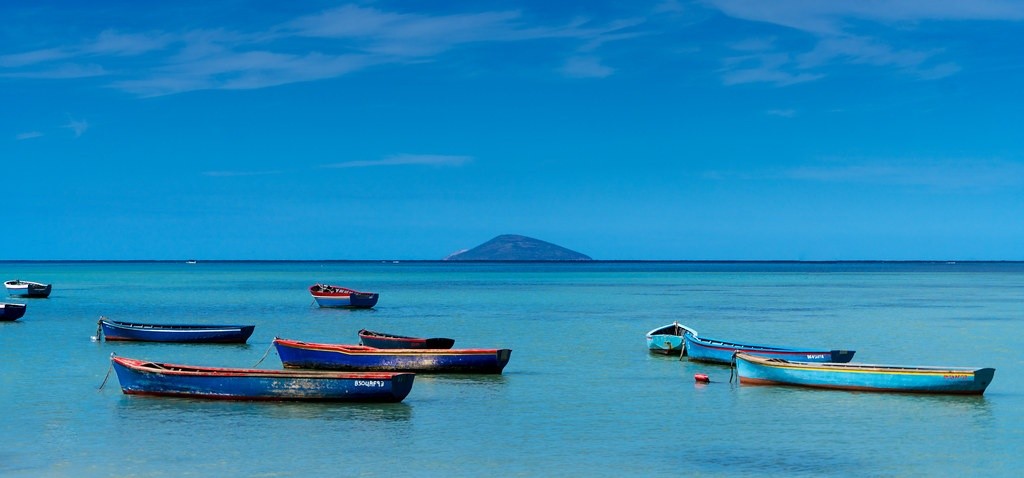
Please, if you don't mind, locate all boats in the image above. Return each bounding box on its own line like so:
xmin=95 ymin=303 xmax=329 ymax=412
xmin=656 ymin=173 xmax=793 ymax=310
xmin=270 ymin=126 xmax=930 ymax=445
xmin=356 ymin=326 xmax=455 ymax=349
xmin=271 ymin=334 xmax=514 ymax=376
xmin=308 ymin=282 xmax=379 ymax=309
xmin=96 ymin=314 xmax=256 ymax=345
xmin=729 ymin=348 xmax=998 ymax=397
xmin=108 ymin=350 xmax=418 ymax=404
xmin=644 ymin=319 xmax=699 ymax=358
xmin=4 ymin=278 xmax=53 ymax=300
xmin=0 ymin=301 xmax=28 ymax=322
xmin=684 ymin=332 xmax=856 ymax=368
